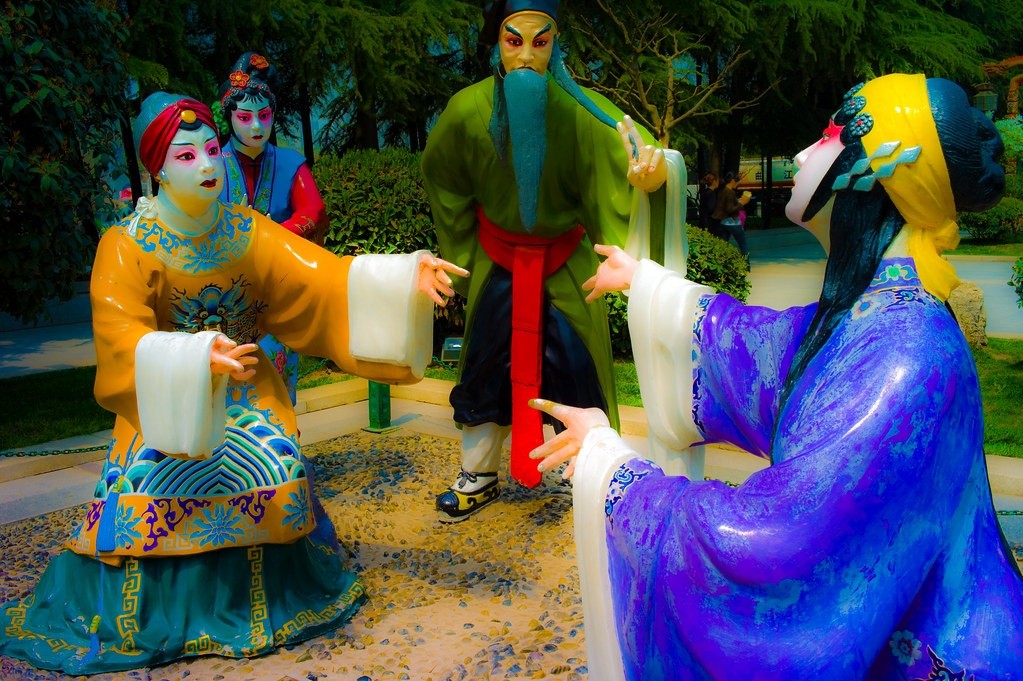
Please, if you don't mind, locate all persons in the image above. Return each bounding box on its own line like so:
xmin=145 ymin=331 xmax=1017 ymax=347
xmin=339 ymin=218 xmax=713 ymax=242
xmin=422 ymin=2 xmax=688 ymax=522
xmin=698 ymin=176 xmax=718 ymax=224
xmin=219 ymin=54 xmax=328 ymax=407
xmin=711 ymin=172 xmax=751 ymax=270
xmin=0 ymin=90 xmax=471 ymax=678
xmin=527 ymin=72 xmax=1023 ymax=681
